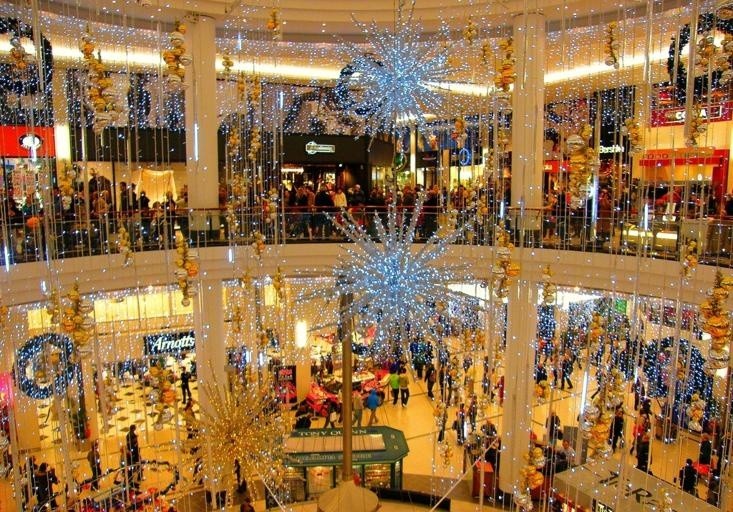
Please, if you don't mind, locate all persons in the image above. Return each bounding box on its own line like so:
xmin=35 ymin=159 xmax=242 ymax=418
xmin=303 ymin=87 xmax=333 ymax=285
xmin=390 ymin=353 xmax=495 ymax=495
xmin=543 ymin=170 xmax=733 ymax=245
xmin=218 ymin=180 xmax=511 ymax=236
xmin=181 ymin=366 xmax=193 ymax=406
xmin=240 ymin=497 xmax=255 ymax=512
xmin=120 ymin=425 xmax=143 ymax=487
xmin=1 ymin=182 xmax=188 ymax=256
xmin=531 ymin=326 xmax=725 ymax=506
xmin=230 ymin=351 xmax=336 ymax=431
xmin=354 ymin=335 xmax=505 ymax=444
xmin=1 ymin=436 xmax=58 ymax=511
xmin=87 ymin=441 xmax=102 ymax=491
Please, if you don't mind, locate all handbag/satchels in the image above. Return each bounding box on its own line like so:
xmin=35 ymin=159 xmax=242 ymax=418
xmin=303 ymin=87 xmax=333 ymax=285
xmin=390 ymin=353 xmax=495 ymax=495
xmin=329 ymin=413 xmax=337 ymax=423
xmin=452 ymin=421 xmax=458 ymax=430
